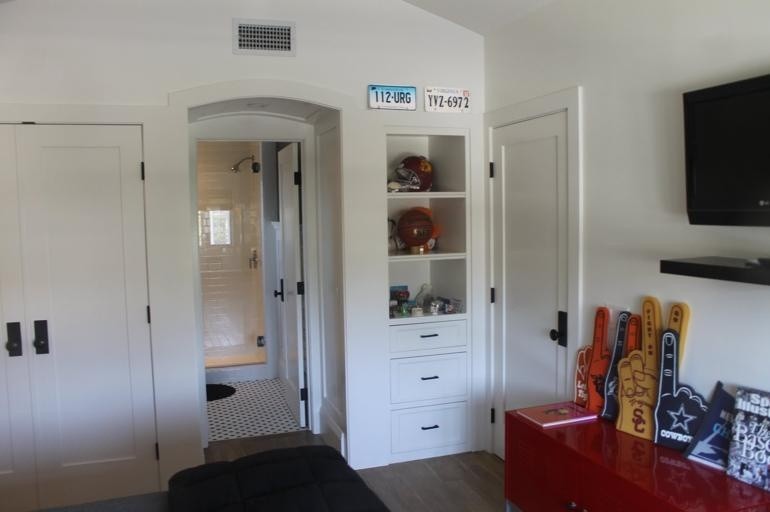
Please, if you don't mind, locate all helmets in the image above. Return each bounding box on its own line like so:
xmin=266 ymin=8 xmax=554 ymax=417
xmin=394 ymin=156 xmax=433 ymax=192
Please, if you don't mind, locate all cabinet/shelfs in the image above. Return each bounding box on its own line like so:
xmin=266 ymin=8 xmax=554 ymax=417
xmin=382 ymin=125 xmax=474 ymax=465
xmin=0 ymin=125 xmax=161 ymax=512
xmin=503 ymin=401 xmax=769 ymax=512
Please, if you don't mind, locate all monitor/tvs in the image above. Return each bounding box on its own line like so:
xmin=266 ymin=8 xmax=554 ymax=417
xmin=682 ymin=74 xmax=770 ymax=226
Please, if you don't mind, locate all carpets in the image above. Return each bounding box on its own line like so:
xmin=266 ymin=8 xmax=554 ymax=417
xmin=207 ymin=383 xmax=237 ymax=401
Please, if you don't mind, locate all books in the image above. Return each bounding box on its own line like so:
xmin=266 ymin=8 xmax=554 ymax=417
xmin=516 ymin=399 xmax=598 ymax=428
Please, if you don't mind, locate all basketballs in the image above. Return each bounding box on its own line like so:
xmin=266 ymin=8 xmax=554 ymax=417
xmin=397 ymin=209 xmax=433 ymax=246
xmin=399 ymin=157 xmax=434 ymax=191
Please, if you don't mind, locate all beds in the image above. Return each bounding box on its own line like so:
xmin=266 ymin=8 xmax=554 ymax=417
xmin=32 ymin=444 xmax=392 ymax=512
